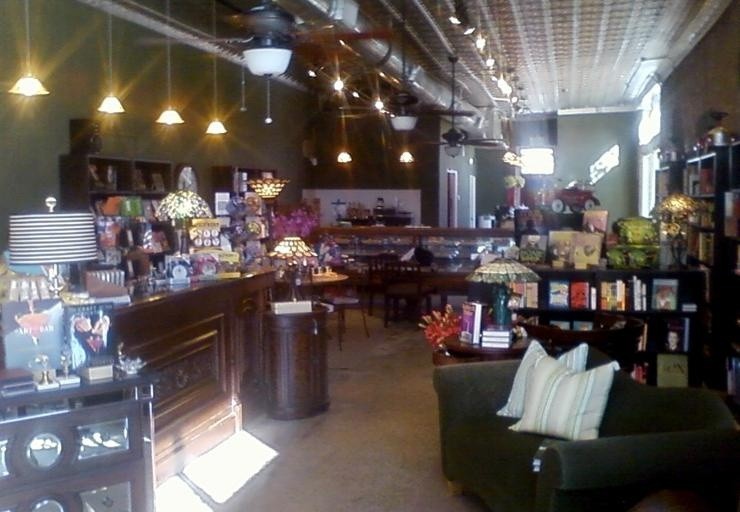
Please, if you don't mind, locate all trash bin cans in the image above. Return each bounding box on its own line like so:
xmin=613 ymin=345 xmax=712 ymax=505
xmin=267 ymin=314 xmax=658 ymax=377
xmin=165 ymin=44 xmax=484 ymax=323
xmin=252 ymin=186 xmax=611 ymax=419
xmin=264 ymin=304 xmax=330 ymax=421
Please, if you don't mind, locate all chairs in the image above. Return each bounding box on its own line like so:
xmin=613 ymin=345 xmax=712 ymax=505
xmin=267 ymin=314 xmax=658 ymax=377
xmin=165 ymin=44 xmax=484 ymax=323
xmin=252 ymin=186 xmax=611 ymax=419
xmin=323 ymin=267 xmax=373 ymax=351
xmin=541 ymin=312 xmax=646 ymax=375
xmin=382 ymin=260 xmax=437 ymax=329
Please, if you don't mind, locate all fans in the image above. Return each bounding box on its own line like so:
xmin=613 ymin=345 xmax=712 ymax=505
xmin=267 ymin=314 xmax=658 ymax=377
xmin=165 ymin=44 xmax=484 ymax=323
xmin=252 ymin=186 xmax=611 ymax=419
xmin=337 ymin=4 xmax=477 ymax=118
xmin=135 ymin=0 xmax=393 ymax=50
xmin=416 ymin=50 xmax=504 ymax=149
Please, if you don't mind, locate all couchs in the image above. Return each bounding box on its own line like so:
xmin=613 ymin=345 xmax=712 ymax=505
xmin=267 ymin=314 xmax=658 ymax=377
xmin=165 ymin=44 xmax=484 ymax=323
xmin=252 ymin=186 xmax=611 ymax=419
xmin=432 ymin=341 xmax=740 ymax=512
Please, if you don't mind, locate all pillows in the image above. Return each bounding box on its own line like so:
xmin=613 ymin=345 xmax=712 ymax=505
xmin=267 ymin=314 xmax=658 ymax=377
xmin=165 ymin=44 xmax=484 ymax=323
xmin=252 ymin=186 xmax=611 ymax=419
xmin=507 ymin=357 xmax=625 ymax=442
xmin=494 ymin=339 xmax=592 ymax=419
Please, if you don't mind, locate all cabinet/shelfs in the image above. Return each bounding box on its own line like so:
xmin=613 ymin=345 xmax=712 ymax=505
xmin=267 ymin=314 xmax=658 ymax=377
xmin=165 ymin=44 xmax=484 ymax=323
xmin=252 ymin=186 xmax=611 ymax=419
xmin=648 ymin=137 xmax=739 ymax=411
xmin=507 ymin=258 xmax=707 ymax=368
xmin=307 ymin=224 xmax=516 ymax=299
xmin=1 ymin=359 xmax=159 ymax=512
xmin=210 ymin=165 xmax=279 ymax=203
xmin=61 ymin=152 xmax=182 ymax=284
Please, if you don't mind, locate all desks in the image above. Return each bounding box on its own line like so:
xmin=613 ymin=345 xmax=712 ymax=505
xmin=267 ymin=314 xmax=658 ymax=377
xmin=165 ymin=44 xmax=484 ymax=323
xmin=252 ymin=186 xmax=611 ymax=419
xmin=270 ymin=265 xmax=351 ymax=299
xmin=446 ymin=322 xmax=554 ymax=363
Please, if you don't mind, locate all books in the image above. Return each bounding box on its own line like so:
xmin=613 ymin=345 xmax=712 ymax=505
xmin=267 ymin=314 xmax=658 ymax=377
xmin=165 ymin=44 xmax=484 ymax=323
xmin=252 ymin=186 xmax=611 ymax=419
xmin=481 ymin=335 xmax=513 ymax=343
xmin=1 ymin=298 xmax=65 ymax=372
xmin=458 ymin=300 xmax=483 ymax=345
xmin=481 ymin=342 xmax=512 ymax=349
xmin=63 ymin=299 xmax=120 ymax=372
xmin=509 ymin=208 xmax=691 ymax=391
xmin=482 ymin=322 xmax=513 ymax=336
xmin=681 ymin=160 xmax=740 ymax=397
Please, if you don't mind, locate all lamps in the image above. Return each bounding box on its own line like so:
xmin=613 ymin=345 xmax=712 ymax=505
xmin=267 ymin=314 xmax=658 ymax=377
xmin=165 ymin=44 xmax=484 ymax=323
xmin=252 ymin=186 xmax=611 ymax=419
xmin=443 ymin=140 xmax=464 ymax=155
xmin=153 ymin=185 xmax=216 ymax=259
xmin=7 ymin=0 xmax=51 ymax=98
xmin=397 ymin=104 xmax=416 ymax=164
xmin=266 ymin=234 xmax=320 ymax=305
xmin=243 ymin=46 xmax=295 ymax=78
xmin=447 ymin=1 xmax=528 ymax=113
xmin=246 ymin=176 xmax=290 ymax=237
xmin=463 ymin=251 xmax=545 ymax=326
xmin=336 ymin=107 xmax=354 ymax=163
xmin=154 ymin=0 xmax=186 ymax=125
xmin=94 ymin=0 xmax=127 ymax=116
xmin=389 ymin=113 xmax=419 ymax=132
xmin=202 ymin=0 xmax=228 ymax=136
xmin=6 ymin=196 xmax=102 ymax=298
